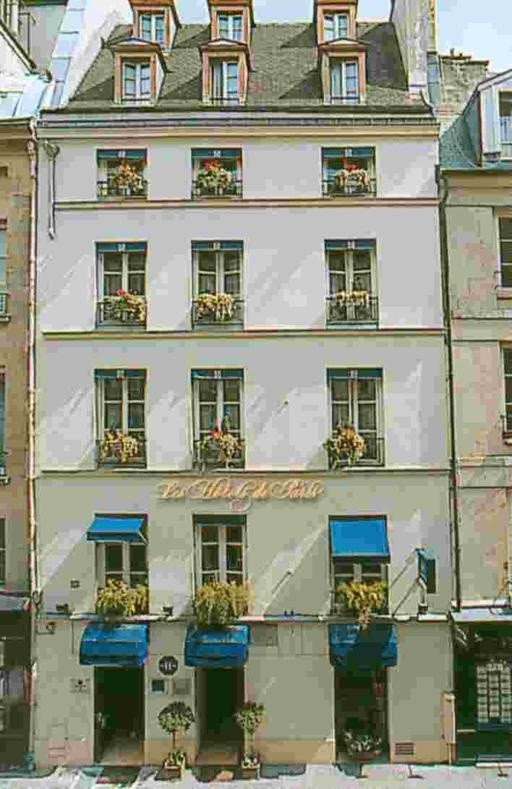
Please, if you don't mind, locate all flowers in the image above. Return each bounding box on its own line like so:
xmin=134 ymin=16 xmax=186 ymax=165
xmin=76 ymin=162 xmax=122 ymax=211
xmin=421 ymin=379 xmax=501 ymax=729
xmin=106 ymin=287 xmax=145 ymax=321
xmin=202 ymin=424 xmax=234 ymax=458
xmin=110 ymin=161 xmax=146 ymax=192
xmin=101 ymin=426 xmax=138 ymax=461
xmin=333 ymin=164 xmax=373 ymax=189
xmin=328 ymin=425 xmax=369 ymax=457
xmin=196 ymin=159 xmax=233 ymax=184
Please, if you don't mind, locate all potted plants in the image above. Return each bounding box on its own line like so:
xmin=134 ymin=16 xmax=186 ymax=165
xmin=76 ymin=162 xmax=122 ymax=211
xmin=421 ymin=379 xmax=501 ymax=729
xmin=336 ymin=726 xmax=385 ymax=762
xmin=156 ymin=705 xmax=195 ymax=778
xmin=230 ymin=695 xmax=265 ymax=778
